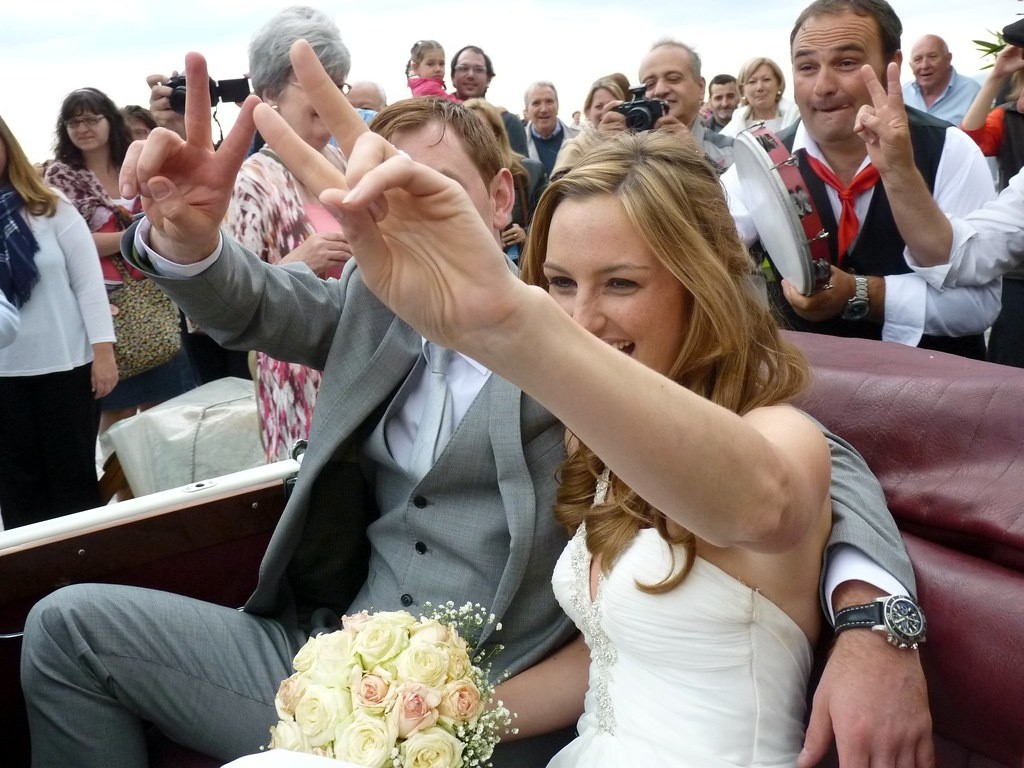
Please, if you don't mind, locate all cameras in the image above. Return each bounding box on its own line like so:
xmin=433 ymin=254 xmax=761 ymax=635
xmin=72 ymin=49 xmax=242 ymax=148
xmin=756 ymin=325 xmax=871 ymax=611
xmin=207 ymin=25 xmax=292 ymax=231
xmin=612 ymin=99 xmax=669 ymax=134
xmin=164 ymin=74 xmax=251 ymax=115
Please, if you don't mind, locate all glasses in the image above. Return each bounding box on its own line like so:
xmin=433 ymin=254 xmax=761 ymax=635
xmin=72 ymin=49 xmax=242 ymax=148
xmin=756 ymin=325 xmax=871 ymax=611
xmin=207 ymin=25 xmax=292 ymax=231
xmin=287 ymin=80 xmax=350 ymax=96
xmin=455 ymin=63 xmax=488 ymax=72
xmin=64 ymin=116 xmax=105 ymax=128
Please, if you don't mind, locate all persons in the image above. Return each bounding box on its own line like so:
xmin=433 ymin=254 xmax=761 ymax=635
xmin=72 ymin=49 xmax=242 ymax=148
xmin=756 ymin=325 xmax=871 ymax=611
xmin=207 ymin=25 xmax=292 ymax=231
xmin=1 ymin=5 xmax=391 ymax=531
xmin=253 ymin=38 xmax=833 ymax=768
xmin=19 ymin=50 xmax=934 ymax=768
xmin=404 ymin=0 xmax=1024 ymax=370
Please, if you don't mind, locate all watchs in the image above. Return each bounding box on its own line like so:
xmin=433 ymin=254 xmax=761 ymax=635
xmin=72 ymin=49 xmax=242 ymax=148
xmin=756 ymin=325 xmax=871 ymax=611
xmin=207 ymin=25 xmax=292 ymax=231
xmin=843 ymin=276 xmax=870 ymax=320
xmin=835 ymin=594 xmax=928 ymax=650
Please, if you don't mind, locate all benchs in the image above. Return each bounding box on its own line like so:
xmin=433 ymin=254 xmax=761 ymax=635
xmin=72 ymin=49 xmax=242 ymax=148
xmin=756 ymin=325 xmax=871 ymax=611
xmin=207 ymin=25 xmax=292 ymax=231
xmin=903 ymin=532 xmax=1024 ymax=768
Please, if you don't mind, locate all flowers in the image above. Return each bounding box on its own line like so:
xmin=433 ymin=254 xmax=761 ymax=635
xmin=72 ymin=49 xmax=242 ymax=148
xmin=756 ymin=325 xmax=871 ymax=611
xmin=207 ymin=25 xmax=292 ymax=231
xmin=260 ymin=597 xmax=519 ymax=768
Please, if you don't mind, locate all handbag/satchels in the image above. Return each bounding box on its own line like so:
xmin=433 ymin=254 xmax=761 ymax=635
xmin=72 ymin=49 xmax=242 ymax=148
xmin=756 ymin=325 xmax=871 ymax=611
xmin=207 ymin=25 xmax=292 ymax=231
xmin=109 ymin=253 xmax=181 ymax=379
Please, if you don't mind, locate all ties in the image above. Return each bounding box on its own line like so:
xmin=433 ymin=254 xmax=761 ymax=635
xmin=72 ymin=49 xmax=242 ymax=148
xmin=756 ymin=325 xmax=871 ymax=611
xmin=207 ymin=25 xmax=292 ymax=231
xmin=805 ymin=150 xmax=880 ymax=264
xmin=410 ymin=343 xmax=454 ymax=480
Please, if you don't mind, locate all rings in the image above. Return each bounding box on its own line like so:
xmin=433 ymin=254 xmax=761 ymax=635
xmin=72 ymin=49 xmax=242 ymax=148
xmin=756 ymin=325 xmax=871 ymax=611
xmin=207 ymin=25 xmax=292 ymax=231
xmin=399 ymin=149 xmax=411 ymax=160
